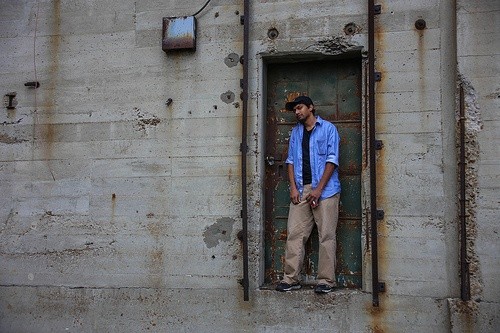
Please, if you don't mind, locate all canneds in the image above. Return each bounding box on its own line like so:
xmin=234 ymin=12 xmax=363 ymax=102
xmin=307 ymin=198 xmax=319 ymax=209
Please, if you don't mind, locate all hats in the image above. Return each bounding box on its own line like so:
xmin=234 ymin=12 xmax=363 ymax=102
xmin=285 ymin=95 xmax=314 ymax=111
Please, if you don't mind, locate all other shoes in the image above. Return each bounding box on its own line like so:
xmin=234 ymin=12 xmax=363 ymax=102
xmin=314 ymin=285 xmax=333 ymax=293
xmin=276 ymin=282 xmax=301 ymax=291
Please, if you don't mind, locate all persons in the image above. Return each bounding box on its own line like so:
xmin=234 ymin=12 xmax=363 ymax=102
xmin=277 ymin=97 xmax=342 ymax=293
